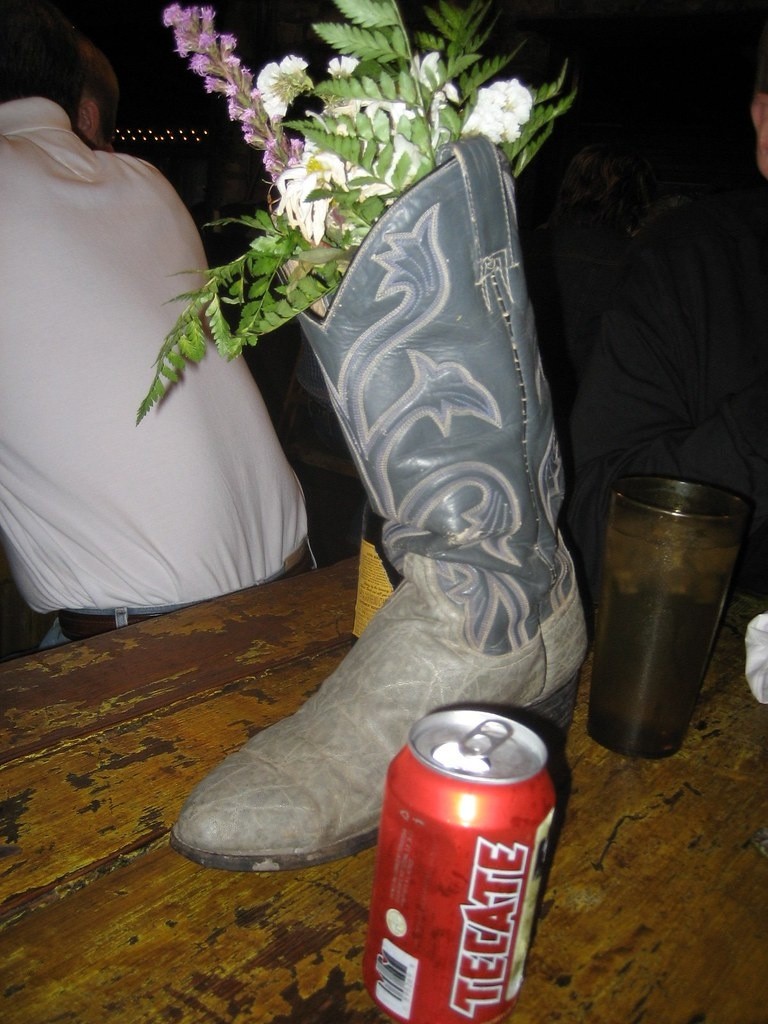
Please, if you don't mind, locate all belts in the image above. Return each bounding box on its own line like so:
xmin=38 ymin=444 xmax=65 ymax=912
xmin=57 ymin=563 xmax=308 ymax=641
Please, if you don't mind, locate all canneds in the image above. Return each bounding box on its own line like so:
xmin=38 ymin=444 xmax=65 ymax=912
xmin=363 ymin=707 xmax=555 ymax=1024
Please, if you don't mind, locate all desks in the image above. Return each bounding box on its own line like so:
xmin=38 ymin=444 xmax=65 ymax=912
xmin=0 ymin=549 xmax=768 ymax=1024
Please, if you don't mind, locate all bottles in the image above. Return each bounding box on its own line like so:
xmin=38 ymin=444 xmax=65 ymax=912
xmin=350 ymin=497 xmax=402 ymax=648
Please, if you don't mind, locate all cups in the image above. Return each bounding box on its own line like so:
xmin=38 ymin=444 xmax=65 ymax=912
xmin=587 ymin=477 xmax=750 ymax=758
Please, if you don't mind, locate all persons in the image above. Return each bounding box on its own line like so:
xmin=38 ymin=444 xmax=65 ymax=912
xmin=72 ymin=40 xmax=123 ymax=154
xmin=0 ymin=10 xmax=318 ymax=651
xmin=527 ymin=52 xmax=768 ymax=604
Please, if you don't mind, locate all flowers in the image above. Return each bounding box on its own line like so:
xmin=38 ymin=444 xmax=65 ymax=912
xmin=135 ymin=0 xmax=569 ymax=417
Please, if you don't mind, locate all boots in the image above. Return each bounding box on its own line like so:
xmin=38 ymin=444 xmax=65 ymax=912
xmin=169 ymin=136 xmax=588 ymax=874
